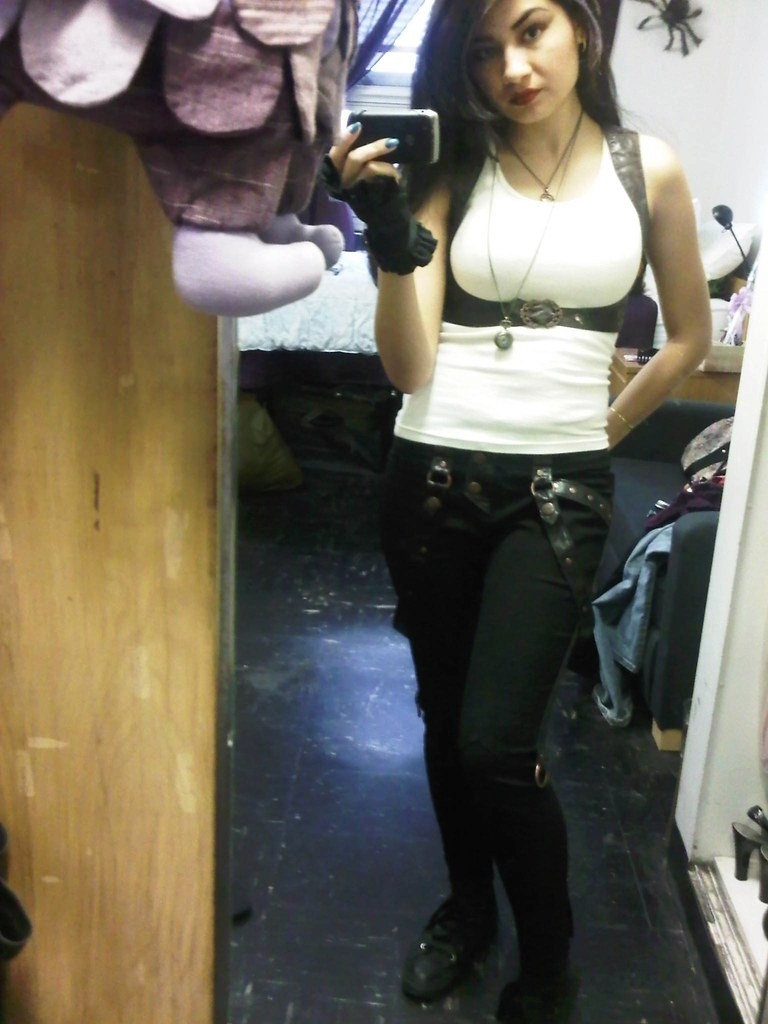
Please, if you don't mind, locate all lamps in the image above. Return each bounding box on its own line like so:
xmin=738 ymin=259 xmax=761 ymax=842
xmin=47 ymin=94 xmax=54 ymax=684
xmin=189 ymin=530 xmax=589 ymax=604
xmin=712 ymin=205 xmax=751 ymax=275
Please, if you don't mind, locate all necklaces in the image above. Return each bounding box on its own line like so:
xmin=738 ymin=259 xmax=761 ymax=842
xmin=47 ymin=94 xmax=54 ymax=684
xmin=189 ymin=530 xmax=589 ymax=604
xmin=487 ymin=110 xmax=585 ymax=349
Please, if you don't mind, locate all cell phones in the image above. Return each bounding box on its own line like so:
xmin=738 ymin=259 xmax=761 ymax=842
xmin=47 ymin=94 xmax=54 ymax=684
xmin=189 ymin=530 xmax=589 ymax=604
xmin=348 ymin=109 xmax=439 ymax=166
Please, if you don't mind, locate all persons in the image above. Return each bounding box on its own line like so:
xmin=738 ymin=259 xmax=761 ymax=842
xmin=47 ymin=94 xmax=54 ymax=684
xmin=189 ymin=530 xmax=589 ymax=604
xmin=326 ymin=0 xmax=712 ymax=1024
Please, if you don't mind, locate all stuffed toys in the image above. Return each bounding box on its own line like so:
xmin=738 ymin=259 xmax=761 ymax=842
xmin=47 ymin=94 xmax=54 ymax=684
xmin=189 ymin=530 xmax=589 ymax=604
xmin=0 ymin=1 xmax=360 ymax=318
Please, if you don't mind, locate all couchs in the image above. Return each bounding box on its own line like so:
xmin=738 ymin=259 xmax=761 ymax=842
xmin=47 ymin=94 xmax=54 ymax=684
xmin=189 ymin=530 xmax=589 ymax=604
xmin=590 ymin=396 xmax=735 ymax=751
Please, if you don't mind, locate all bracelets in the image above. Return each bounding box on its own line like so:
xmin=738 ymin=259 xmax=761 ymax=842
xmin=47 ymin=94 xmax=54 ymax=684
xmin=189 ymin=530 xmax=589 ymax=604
xmin=611 ymin=407 xmax=634 ymax=430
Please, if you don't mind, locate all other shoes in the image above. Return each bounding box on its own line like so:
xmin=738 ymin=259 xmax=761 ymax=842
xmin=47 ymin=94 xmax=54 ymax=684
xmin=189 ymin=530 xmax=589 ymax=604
xmin=400 ymin=900 xmax=472 ymax=1001
xmin=495 ymin=966 xmax=568 ymax=1024
